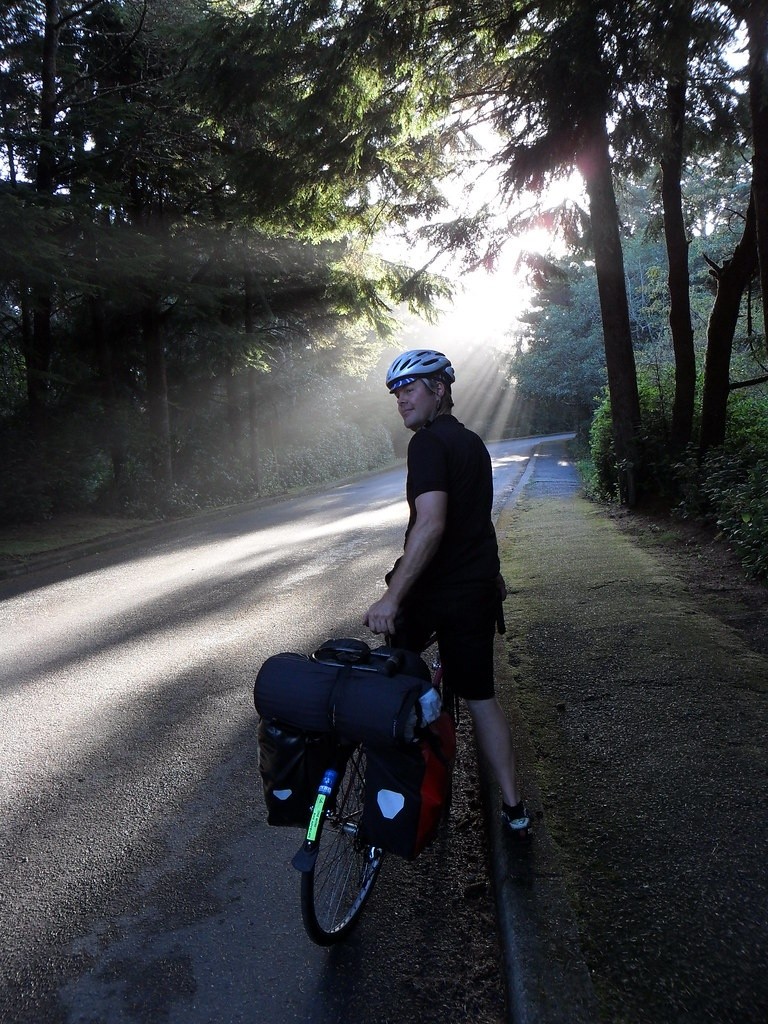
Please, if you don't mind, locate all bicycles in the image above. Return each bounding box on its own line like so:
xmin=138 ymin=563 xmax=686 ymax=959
xmin=289 ymin=596 xmax=461 ymax=948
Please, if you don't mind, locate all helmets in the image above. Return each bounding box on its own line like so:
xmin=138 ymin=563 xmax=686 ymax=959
xmin=386 ymin=349 xmax=455 ymax=394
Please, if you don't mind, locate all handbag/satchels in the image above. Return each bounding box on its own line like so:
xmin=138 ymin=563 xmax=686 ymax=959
xmin=256 ymin=717 xmax=337 ymax=829
xmin=360 ymin=681 xmax=456 ymax=862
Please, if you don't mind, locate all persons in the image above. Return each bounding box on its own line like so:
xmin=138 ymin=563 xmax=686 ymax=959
xmin=362 ymin=349 xmax=530 ymax=829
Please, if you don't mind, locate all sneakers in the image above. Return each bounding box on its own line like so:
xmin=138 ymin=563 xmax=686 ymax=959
xmin=497 ymin=805 xmax=530 ymax=837
